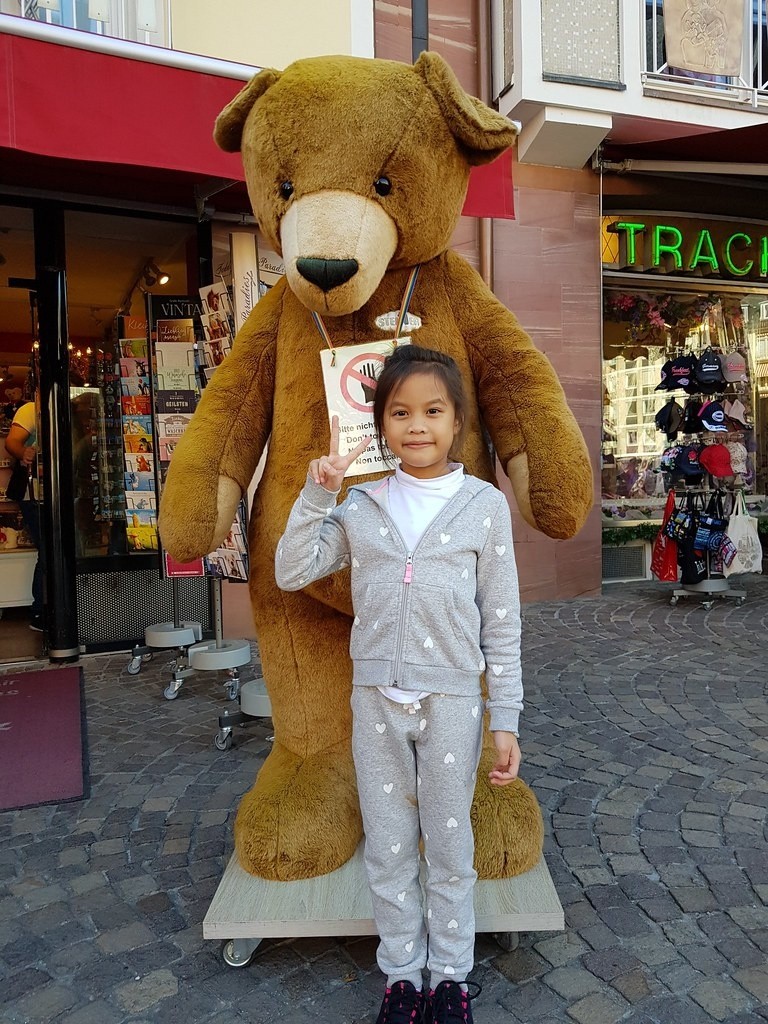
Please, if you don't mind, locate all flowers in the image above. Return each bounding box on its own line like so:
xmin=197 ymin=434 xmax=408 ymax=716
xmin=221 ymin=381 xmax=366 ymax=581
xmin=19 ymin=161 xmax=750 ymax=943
xmin=602 ymin=287 xmax=744 ymax=356
xmin=601 ymin=500 xmax=768 ymax=547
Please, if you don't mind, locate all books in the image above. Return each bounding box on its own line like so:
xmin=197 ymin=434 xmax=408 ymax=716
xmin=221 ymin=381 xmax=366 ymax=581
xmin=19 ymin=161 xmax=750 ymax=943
xmin=119 ymin=281 xmax=251 ymax=582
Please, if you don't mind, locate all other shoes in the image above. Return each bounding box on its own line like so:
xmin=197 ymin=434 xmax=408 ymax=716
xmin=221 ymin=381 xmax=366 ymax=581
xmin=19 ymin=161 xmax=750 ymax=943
xmin=28 ymin=615 xmax=45 ymax=632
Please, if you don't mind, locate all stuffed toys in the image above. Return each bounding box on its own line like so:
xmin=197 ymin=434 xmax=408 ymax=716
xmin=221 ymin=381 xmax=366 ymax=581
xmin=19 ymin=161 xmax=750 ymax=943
xmin=158 ymin=53 xmax=597 ymax=880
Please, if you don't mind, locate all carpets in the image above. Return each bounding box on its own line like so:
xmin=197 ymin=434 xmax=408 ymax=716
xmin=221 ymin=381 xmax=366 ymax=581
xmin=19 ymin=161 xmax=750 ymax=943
xmin=1 ymin=665 xmax=91 ymax=813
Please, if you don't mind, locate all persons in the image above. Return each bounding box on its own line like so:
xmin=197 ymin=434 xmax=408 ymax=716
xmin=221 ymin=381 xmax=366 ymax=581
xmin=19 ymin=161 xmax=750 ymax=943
xmin=4 ymin=370 xmax=100 ymax=632
xmin=275 ymin=344 xmax=524 ymax=1024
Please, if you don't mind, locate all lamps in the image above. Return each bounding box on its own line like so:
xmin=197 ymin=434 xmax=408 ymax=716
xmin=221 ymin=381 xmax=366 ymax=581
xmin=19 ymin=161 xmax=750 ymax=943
xmin=150 ymin=261 xmax=170 ymax=286
xmin=2 ymin=369 xmax=13 ymax=381
xmin=143 ymin=269 xmax=156 ymax=286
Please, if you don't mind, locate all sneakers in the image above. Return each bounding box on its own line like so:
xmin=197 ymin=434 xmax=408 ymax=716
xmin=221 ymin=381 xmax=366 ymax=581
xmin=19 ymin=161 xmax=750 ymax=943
xmin=377 ymin=974 xmax=426 ymax=1024
xmin=425 ymin=980 xmax=482 ymax=1024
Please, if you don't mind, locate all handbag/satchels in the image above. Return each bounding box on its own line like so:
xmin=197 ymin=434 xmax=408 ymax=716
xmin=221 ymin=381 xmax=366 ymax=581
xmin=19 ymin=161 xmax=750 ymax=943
xmin=650 ymin=488 xmax=763 ymax=585
xmin=644 ymin=456 xmax=665 ymax=497
xmin=6 ymin=459 xmax=29 ymax=503
xmin=615 ymin=459 xmax=648 ymax=498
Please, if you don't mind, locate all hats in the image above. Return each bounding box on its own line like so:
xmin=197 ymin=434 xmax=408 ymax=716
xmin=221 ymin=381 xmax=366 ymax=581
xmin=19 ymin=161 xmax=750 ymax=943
xmin=653 ymin=347 xmax=757 ymax=494
